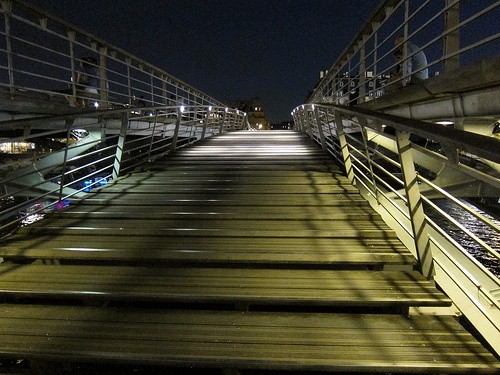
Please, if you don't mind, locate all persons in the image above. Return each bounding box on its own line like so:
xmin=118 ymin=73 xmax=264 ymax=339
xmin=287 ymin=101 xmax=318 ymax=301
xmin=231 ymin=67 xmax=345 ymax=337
xmin=383 ymin=35 xmax=429 ymax=93
xmin=138 ymin=94 xmax=146 ymax=116
xmin=170 ymin=92 xmax=210 ymax=122
xmin=76 ymin=56 xmax=100 ymax=111
